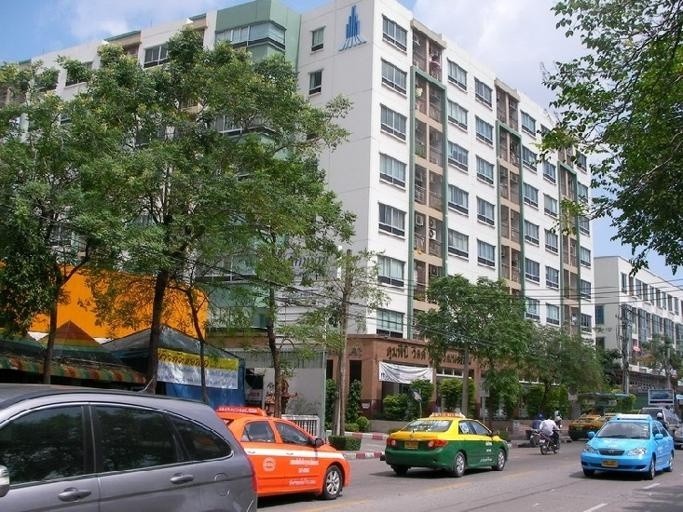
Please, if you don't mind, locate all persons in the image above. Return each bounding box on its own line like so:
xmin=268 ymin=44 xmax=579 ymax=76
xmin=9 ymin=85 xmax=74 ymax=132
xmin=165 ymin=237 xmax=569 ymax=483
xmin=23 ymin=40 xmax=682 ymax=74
xmin=427 ymin=401 xmax=441 ymax=416
xmin=532 ymin=413 xmax=543 ymax=429
xmin=657 ymin=412 xmax=669 ymax=432
xmin=539 ymin=415 xmax=559 ymax=448
xmin=554 ymin=407 xmax=562 ymax=427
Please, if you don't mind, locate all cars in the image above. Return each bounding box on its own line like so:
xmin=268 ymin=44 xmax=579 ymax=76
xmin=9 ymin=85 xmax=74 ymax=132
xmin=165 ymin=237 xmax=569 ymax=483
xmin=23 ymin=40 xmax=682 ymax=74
xmin=0 ymin=384 xmax=258 ymax=512
xmin=384 ymin=412 xmax=509 ymax=477
xmin=215 ymin=406 xmax=351 ymax=499
xmin=569 ymin=408 xmax=683 ymax=480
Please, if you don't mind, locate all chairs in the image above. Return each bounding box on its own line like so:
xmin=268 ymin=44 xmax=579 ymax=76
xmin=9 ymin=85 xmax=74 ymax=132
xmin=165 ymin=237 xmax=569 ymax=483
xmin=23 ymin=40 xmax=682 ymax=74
xmin=249 ymin=424 xmax=270 ymax=440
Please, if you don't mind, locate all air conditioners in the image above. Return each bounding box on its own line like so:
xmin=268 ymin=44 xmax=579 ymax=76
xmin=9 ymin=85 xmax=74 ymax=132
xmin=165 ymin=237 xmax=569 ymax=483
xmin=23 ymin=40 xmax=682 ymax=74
xmin=415 ymin=214 xmax=437 ymax=241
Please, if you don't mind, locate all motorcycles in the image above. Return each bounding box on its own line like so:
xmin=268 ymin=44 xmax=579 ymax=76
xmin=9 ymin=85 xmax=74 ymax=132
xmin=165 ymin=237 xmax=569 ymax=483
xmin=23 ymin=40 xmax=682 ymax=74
xmin=528 ymin=430 xmax=560 ymax=455
